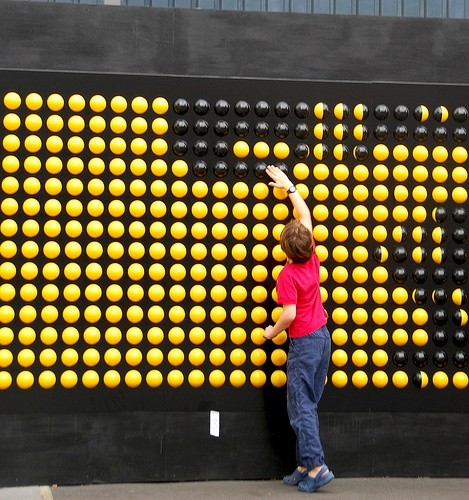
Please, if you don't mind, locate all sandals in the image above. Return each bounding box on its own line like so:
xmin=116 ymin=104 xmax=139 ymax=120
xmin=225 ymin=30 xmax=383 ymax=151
xmin=283 ymin=468 xmax=308 ymax=485
xmin=298 ymin=465 xmax=334 ymax=492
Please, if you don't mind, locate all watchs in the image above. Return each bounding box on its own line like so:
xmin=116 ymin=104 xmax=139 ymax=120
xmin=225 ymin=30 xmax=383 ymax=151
xmin=287 ymin=186 xmax=297 ymax=194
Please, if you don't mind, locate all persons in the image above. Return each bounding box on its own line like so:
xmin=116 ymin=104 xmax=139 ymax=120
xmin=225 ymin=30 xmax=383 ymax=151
xmin=261 ymin=164 xmax=335 ymax=493
